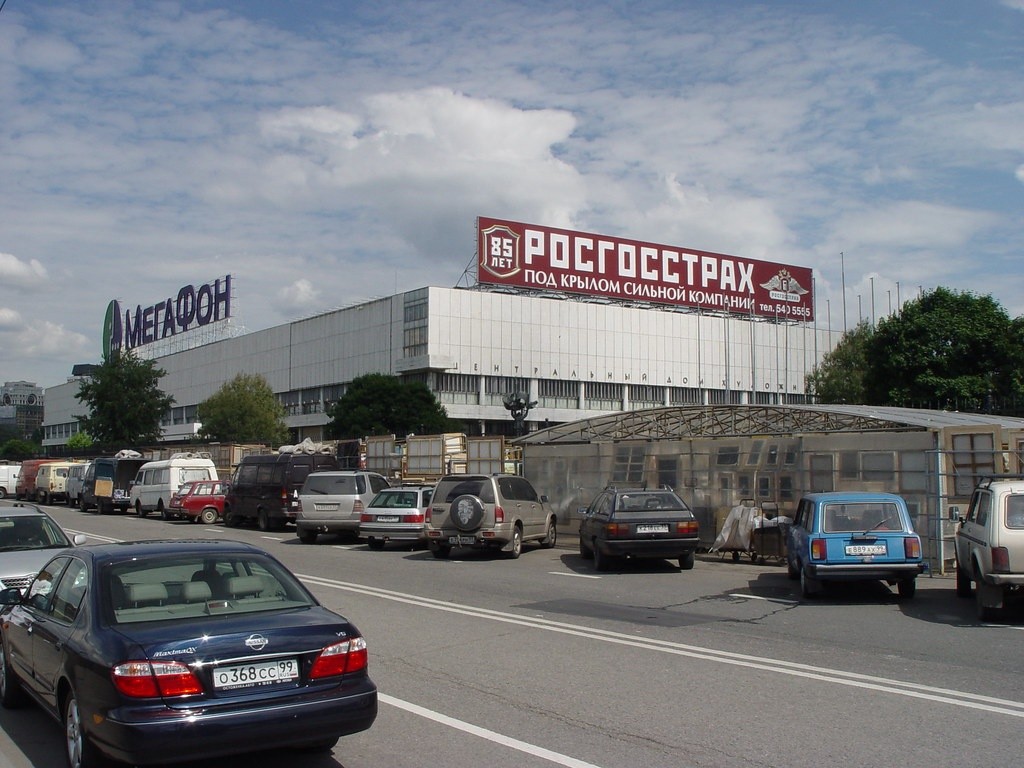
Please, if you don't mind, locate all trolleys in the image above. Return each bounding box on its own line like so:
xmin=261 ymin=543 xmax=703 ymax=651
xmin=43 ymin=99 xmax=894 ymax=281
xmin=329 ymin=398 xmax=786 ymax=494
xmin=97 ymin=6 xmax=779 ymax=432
xmin=752 ymin=500 xmax=792 ymax=565
xmin=731 ymin=498 xmax=761 ymax=562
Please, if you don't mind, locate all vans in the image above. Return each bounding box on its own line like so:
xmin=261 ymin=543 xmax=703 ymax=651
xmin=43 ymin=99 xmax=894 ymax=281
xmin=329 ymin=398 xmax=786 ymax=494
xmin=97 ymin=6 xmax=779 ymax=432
xmin=0 ymin=460 xmax=24 ymax=499
xmin=35 ymin=462 xmax=83 ymax=505
xmin=129 ymin=457 xmax=221 ymax=520
xmin=13 ymin=459 xmax=65 ymax=502
xmin=222 ymin=452 xmax=338 ymax=532
xmin=62 ymin=463 xmax=93 ymax=508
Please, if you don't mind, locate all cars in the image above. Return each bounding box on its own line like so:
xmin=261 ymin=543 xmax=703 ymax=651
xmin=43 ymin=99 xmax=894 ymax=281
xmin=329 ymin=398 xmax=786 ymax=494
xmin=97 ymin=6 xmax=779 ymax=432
xmin=168 ymin=480 xmax=233 ymax=526
xmin=0 ymin=539 xmax=379 ymax=768
xmin=787 ymin=491 xmax=930 ymax=598
xmin=358 ymin=483 xmax=437 ymax=548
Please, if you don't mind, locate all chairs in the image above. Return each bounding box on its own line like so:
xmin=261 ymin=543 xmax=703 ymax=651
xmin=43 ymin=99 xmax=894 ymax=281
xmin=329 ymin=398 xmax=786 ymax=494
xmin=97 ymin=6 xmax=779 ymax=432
xmin=861 ymin=509 xmax=882 ymax=530
xmin=825 ymin=510 xmax=837 ymax=531
xmin=111 ymin=575 xmax=125 ymax=610
xmin=191 ymin=569 xmax=223 ymax=601
xmin=644 ymin=497 xmax=662 ymax=510
xmin=129 ymin=583 xmax=168 ymax=607
xmin=179 ymin=581 xmax=212 ymax=605
xmin=225 ymin=576 xmax=264 ymax=599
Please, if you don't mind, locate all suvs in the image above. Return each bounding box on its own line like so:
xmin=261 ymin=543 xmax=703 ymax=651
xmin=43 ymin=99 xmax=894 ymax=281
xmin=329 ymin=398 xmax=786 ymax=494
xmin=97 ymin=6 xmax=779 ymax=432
xmin=423 ymin=472 xmax=557 ymax=559
xmin=577 ymin=485 xmax=699 ymax=569
xmin=295 ymin=470 xmax=391 ymax=542
xmin=77 ymin=459 xmax=155 ymax=514
xmin=1 ymin=503 xmax=87 ymax=614
xmin=947 ymin=476 xmax=1024 ymax=620
